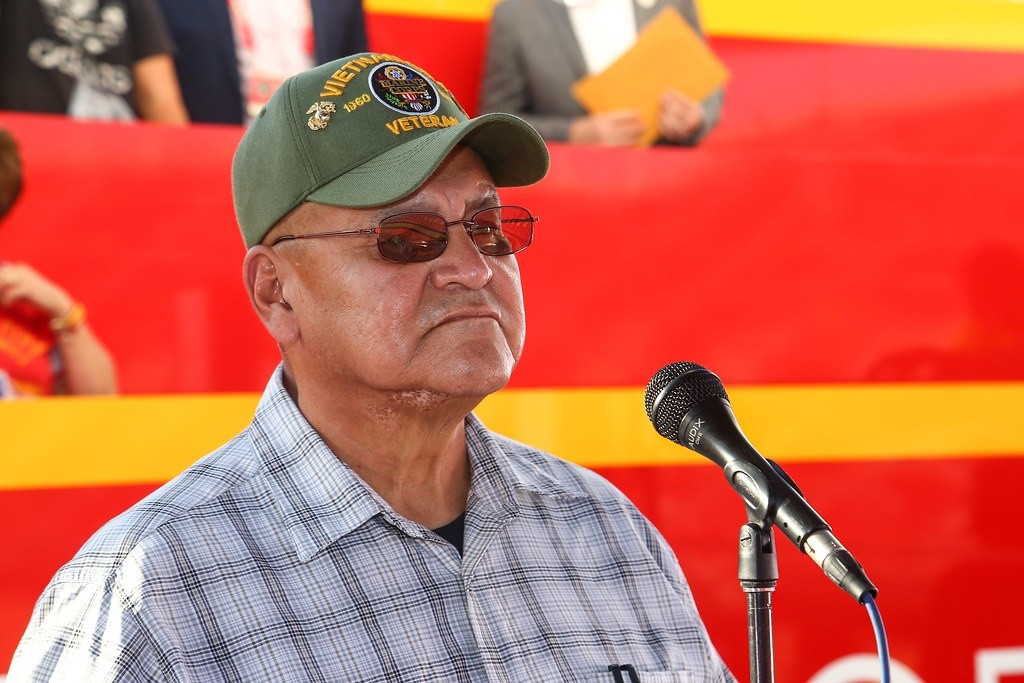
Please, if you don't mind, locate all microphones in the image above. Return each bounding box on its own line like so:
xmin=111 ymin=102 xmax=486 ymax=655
xmin=644 ymin=361 xmax=880 ymax=605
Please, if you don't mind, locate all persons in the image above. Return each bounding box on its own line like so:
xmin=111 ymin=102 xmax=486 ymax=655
xmin=0 ymin=0 xmax=370 ymax=124
xmin=5 ymin=53 xmax=740 ymax=683
xmin=0 ymin=126 xmax=118 ymax=398
xmin=480 ymin=0 xmax=725 ymax=147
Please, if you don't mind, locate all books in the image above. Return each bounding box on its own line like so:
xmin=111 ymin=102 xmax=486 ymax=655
xmin=572 ymin=3 xmax=731 ymax=145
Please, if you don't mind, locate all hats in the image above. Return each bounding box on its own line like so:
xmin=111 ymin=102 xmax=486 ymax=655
xmin=231 ymin=52 xmax=550 ymax=251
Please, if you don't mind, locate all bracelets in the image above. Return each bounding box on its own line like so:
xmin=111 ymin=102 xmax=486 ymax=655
xmin=50 ymin=303 xmax=86 ymax=334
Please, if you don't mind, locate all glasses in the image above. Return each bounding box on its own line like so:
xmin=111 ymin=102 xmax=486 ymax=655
xmin=271 ymin=206 xmax=539 ymax=264
